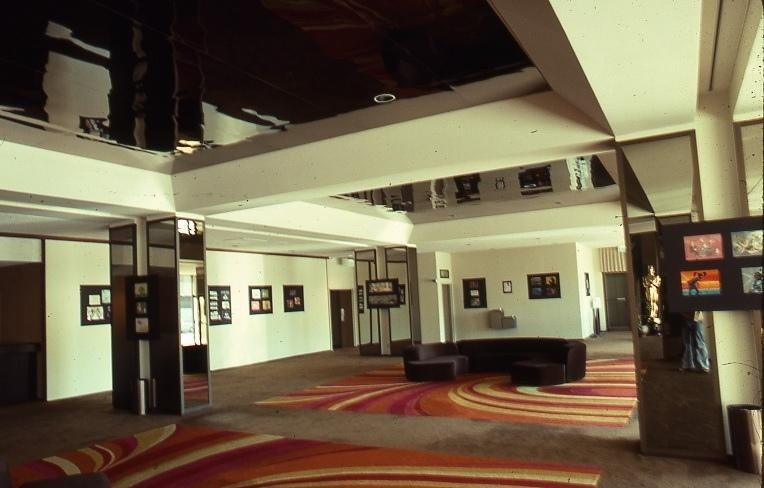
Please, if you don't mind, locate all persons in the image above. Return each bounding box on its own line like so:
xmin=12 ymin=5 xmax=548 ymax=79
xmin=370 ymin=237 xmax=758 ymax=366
xmin=642 ymin=264 xmax=661 ymax=318
xmin=687 ymin=271 xmax=707 ymax=295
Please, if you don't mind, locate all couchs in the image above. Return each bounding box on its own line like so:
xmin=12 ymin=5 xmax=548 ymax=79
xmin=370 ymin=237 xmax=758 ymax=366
xmin=402 ymin=336 xmax=586 ymax=386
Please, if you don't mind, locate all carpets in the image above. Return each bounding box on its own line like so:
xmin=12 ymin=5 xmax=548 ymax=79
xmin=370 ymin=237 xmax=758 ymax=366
xmin=256 ymin=352 xmax=638 ymax=426
xmin=1 ymin=423 xmax=605 ymax=486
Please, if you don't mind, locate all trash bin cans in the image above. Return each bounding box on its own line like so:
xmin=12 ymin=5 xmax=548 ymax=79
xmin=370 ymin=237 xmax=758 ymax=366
xmin=0 ymin=343 xmax=41 ymax=405
xmin=727 ymin=403 xmax=763 ymax=475
xmin=137 ymin=378 xmax=149 ymax=416
